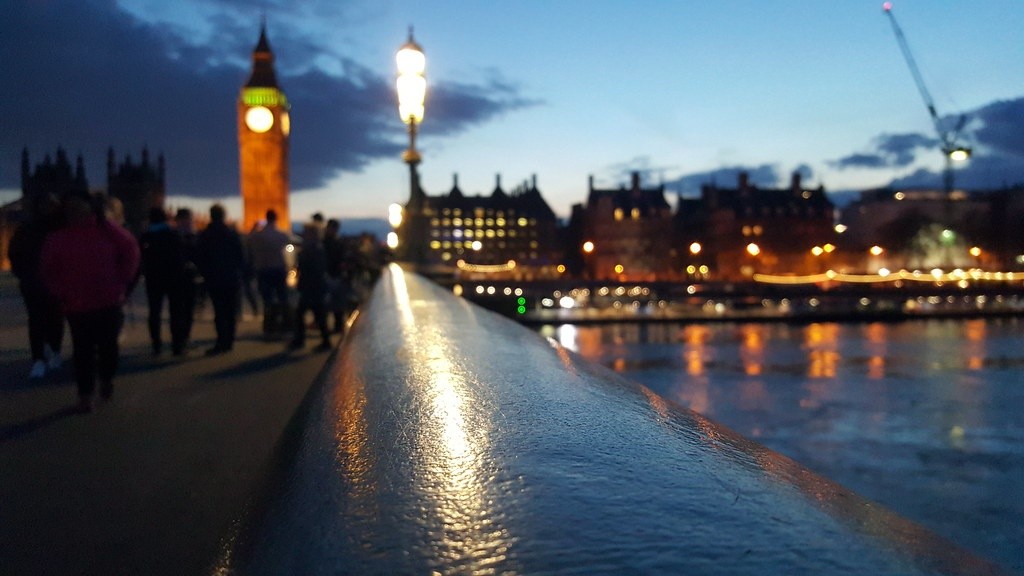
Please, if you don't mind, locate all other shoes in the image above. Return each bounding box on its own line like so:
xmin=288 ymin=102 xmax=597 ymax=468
xmin=207 ymin=344 xmax=232 ymax=356
xmin=313 ymin=340 xmax=330 ymax=352
xmin=171 ymin=342 xmax=185 ymax=354
xmin=95 ymin=384 xmax=113 ymax=401
xmin=288 ymin=338 xmax=304 ymax=346
xmin=76 ymin=399 xmax=97 ymax=414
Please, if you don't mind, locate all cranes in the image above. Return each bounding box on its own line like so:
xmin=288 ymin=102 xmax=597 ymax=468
xmin=885 ymin=5 xmax=972 ymax=194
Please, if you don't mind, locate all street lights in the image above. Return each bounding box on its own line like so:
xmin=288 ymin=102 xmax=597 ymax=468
xmin=394 ymin=24 xmax=429 ymax=270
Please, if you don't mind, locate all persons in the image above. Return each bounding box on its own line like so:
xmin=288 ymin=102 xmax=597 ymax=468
xmin=7 ymin=188 xmax=393 ymax=400
